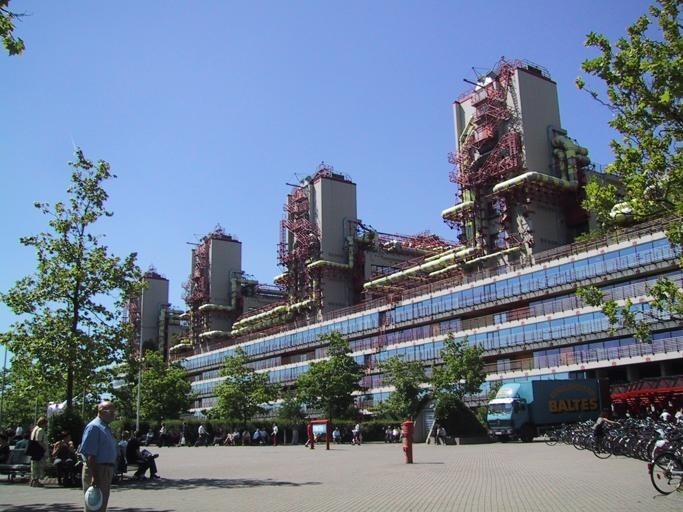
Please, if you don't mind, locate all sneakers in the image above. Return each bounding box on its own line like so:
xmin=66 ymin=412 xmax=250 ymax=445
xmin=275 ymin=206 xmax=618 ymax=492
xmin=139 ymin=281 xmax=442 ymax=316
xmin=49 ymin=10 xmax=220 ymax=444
xmin=30 ymin=480 xmax=43 ymax=487
xmin=151 ymin=474 xmax=160 ymax=478
xmin=134 ymin=471 xmax=147 ymax=480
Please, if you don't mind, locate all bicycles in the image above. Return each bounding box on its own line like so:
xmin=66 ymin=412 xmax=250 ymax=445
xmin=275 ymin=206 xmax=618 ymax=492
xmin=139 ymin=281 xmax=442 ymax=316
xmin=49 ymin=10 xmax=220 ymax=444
xmin=544 ymin=416 xmax=682 ymax=494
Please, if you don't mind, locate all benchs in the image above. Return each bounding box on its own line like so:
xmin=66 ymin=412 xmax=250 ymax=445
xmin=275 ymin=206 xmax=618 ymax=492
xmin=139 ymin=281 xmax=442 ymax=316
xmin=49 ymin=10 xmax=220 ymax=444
xmin=0 ymin=449 xmax=61 ymax=484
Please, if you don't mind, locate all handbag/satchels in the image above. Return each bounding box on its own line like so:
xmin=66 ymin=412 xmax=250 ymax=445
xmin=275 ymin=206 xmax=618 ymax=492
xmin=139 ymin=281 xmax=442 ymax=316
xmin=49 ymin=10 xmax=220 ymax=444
xmin=26 ymin=440 xmax=42 ymax=456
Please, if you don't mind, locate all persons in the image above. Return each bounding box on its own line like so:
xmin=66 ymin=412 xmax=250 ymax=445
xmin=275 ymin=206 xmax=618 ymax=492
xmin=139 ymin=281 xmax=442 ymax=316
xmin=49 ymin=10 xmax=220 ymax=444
xmin=436 ymin=424 xmax=448 ymax=446
xmin=591 ymin=406 xmax=683 ymax=453
xmin=0 ymin=401 xmax=167 ymax=511
xmin=195 ymin=422 xmax=403 ymax=448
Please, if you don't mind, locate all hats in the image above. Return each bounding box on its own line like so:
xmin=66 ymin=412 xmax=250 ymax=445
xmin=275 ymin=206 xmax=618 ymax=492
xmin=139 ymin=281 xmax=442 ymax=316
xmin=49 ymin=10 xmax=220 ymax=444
xmin=85 ymin=486 xmax=103 ymax=511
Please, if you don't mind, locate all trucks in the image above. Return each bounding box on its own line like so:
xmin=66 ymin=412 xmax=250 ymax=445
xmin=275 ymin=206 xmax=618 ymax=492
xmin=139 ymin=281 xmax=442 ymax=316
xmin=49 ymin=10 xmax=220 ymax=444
xmin=485 ymin=380 xmax=601 ymax=442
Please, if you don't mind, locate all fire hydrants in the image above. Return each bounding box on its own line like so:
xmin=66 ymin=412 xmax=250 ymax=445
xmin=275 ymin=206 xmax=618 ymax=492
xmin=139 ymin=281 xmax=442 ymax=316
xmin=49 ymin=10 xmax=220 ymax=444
xmin=401 ymin=419 xmax=416 ymax=464
